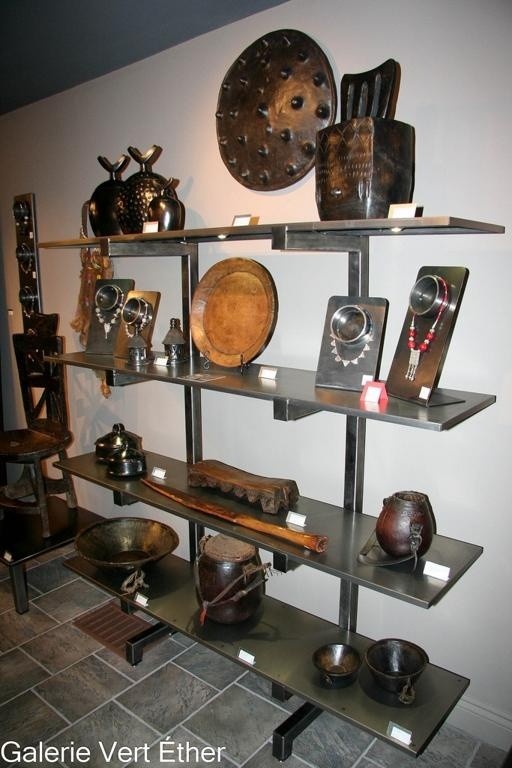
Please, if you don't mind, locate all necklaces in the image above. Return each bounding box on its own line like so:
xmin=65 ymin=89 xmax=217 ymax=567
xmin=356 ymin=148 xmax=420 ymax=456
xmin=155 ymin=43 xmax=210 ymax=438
xmin=120 ymin=295 xmax=149 ymax=338
xmin=95 ymin=283 xmax=124 ymax=339
xmin=404 ymin=273 xmax=449 ymax=381
xmin=331 ymin=302 xmax=373 ymax=367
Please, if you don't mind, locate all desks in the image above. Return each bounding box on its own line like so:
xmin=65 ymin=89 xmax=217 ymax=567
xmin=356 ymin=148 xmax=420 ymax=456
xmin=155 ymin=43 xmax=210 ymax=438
xmin=0 ymin=495 xmax=107 ymax=616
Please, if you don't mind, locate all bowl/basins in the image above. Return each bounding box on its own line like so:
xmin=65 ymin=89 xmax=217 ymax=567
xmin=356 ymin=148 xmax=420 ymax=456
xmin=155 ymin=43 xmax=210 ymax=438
xmin=312 ymin=636 xmax=430 ymax=692
xmin=73 ymin=515 xmax=179 ymax=572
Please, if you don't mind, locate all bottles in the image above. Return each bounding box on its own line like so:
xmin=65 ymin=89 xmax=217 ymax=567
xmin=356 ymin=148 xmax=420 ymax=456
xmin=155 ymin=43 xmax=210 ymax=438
xmin=377 ymin=490 xmax=433 ymax=558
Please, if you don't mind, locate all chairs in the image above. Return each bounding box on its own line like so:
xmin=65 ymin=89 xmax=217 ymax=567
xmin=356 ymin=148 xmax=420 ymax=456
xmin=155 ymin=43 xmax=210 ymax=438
xmin=0 ymin=333 xmax=79 ymax=540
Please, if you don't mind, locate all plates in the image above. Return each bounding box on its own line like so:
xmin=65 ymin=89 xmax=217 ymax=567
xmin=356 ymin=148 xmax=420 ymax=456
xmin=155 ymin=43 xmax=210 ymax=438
xmin=190 ymin=258 xmax=276 ymax=368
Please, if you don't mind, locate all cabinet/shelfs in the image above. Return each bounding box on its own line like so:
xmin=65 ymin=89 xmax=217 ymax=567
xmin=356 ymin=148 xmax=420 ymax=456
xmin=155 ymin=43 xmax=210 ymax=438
xmin=39 ymin=215 xmax=506 ymax=762
